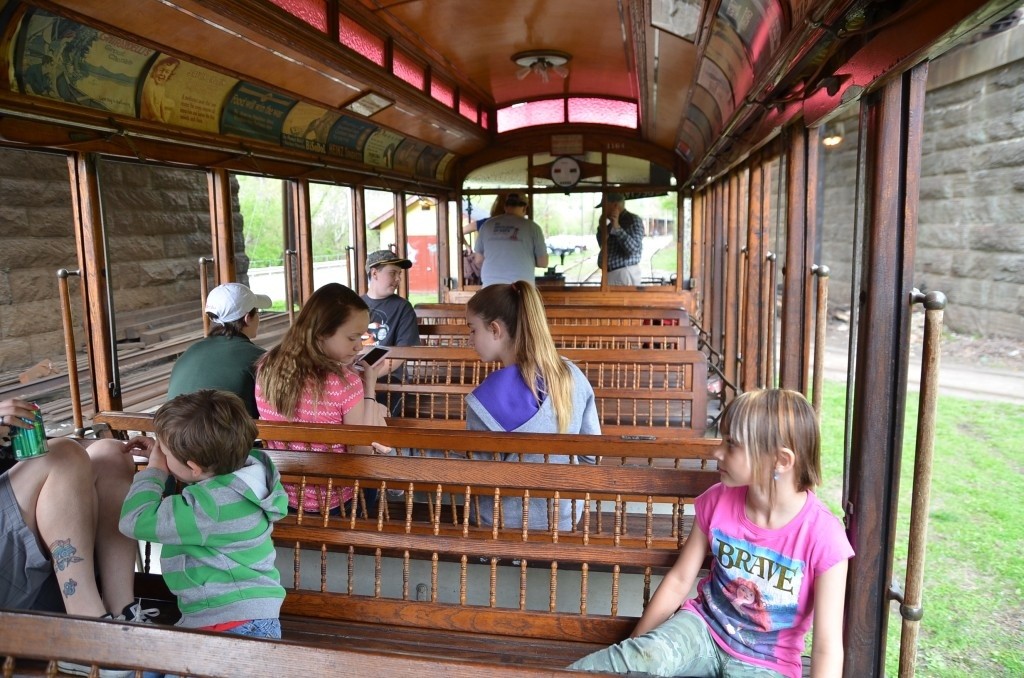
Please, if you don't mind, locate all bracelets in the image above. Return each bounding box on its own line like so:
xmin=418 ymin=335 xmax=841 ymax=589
xmin=364 ymin=397 xmax=376 ymax=402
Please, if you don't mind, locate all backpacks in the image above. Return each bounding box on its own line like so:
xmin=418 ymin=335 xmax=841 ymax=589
xmin=640 ymin=304 xmax=684 ymax=353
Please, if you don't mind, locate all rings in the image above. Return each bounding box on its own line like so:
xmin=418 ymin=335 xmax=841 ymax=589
xmin=0 ymin=415 xmax=5 ymax=426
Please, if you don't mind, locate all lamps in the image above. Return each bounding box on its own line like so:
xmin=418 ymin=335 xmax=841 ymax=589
xmin=509 ymin=50 xmax=572 ymax=85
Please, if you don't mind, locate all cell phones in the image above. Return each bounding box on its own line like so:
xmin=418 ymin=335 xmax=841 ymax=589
xmin=352 ymin=345 xmax=391 ymax=373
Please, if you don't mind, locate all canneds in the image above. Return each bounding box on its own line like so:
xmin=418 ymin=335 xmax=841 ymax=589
xmin=6 ymin=401 xmax=50 ymax=462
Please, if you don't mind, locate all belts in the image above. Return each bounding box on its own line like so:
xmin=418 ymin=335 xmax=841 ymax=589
xmin=605 ymin=261 xmax=637 ymax=273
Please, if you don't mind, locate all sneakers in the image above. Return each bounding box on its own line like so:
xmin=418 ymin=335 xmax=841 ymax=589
xmin=107 ymin=597 xmax=168 ymax=677
xmin=55 ymin=609 xmax=137 ymax=677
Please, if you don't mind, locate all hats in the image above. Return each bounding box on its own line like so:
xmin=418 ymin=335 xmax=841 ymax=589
xmin=205 ymin=283 xmax=274 ymax=326
xmin=594 ymin=189 xmax=625 ymax=209
xmin=506 ymin=188 xmax=531 ymax=216
xmin=364 ymin=249 xmax=412 ymax=277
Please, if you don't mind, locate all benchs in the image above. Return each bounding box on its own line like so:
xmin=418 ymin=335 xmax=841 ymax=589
xmin=1 ymin=274 xmax=724 ymax=678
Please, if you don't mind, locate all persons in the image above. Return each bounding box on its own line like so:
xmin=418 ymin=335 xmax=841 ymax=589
xmin=350 ymin=249 xmax=420 ymax=498
xmin=463 ymin=190 xmax=509 ymax=256
xmin=167 ymin=281 xmax=268 ymax=449
xmin=372 ymin=279 xmax=605 ymax=532
xmin=473 ymin=191 xmax=548 ymax=291
xmin=594 ymin=193 xmax=645 ymax=291
xmin=0 ymin=396 xmax=165 ymax=677
xmin=118 ymin=390 xmax=289 ymax=678
xmin=565 ymin=387 xmax=856 ymax=678
xmin=253 ymin=282 xmax=379 ymax=519
